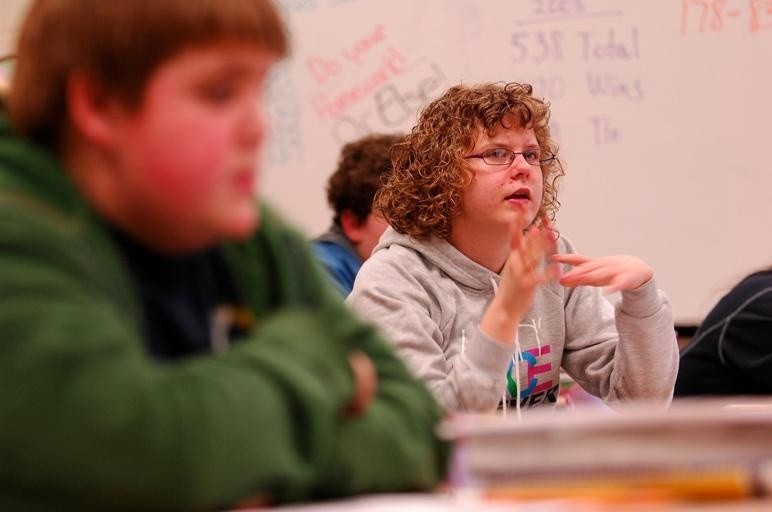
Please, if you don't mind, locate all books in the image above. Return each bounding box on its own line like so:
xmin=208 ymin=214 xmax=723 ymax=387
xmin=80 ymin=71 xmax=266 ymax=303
xmin=434 ymin=391 xmax=772 ymax=484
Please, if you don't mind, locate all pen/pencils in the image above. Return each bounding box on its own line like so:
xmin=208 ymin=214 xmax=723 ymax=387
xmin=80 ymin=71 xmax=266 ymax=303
xmin=479 ymin=459 xmax=772 ymax=506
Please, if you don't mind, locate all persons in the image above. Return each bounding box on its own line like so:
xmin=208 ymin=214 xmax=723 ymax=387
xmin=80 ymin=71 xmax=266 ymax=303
xmin=297 ymin=134 xmax=410 ymax=301
xmin=0 ymin=0 xmax=456 ymax=511
xmin=339 ymin=82 xmax=682 ymax=415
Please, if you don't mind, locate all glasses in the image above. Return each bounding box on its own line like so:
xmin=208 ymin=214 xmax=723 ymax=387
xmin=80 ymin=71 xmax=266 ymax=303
xmin=460 ymin=145 xmax=557 ymax=169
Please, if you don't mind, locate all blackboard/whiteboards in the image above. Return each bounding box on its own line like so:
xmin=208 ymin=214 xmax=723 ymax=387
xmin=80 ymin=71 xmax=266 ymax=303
xmin=0 ymin=0 xmax=772 ymax=338
